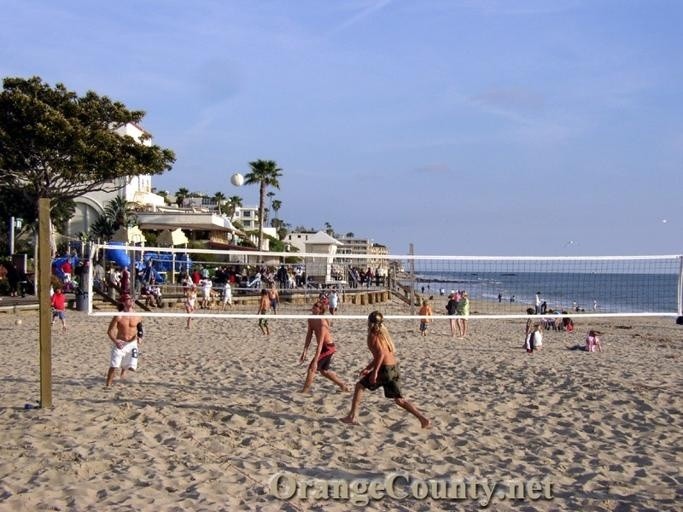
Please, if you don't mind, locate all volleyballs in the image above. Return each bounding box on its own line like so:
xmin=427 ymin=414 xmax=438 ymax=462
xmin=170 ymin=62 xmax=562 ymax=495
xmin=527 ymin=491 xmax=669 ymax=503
xmin=231 ymin=174 xmax=244 ymax=186
xmin=15 ymin=319 xmax=22 ymax=326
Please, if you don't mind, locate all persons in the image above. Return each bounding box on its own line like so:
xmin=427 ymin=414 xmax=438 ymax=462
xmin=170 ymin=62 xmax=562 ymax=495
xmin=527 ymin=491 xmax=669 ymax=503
xmin=103 ymin=292 xmax=144 ymax=389
xmin=341 ymin=311 xmax=427 ymax=428
xmin=298 ymin=299 xmax=350 ymax=396
